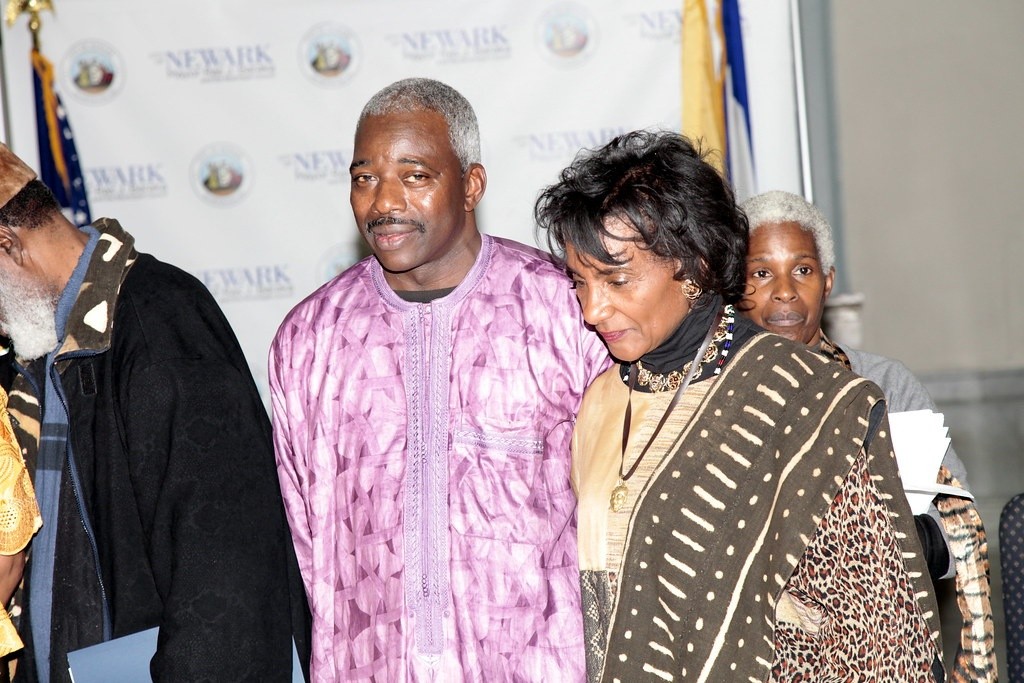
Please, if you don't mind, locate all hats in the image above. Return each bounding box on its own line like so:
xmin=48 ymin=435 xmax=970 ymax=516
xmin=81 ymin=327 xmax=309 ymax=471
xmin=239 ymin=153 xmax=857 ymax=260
xmin=0 ymin=140 xmax=38 ymax=209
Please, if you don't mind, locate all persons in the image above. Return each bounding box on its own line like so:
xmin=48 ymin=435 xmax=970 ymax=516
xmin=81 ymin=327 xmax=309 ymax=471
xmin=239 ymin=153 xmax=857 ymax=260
xmin=0 ymin=142 xmax=312 ymax=683
xmin=266 ymin=77 xmax=618 ymax=683
xmin=528 ymin=125 xmax=952 ymax=683
xmin=0 ymin=383 xmax=43 ymax=683
xmin=722 ymin=188 xmax=976 ymax=683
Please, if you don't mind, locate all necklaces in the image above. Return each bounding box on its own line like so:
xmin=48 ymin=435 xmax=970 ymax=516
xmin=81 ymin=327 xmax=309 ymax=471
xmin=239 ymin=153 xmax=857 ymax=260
xmin=627 ymin=311 xmax=735 ymax=395
xmin=609 ymin=307 xmax=731 ymax=512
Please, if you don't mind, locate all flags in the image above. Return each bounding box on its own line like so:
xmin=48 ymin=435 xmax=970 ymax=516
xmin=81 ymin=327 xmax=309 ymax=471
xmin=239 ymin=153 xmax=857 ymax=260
xmin=31 ymin=46 xmax=93 ymax=232
xmin=679 ymin=1 xmax=758 ymax=200
